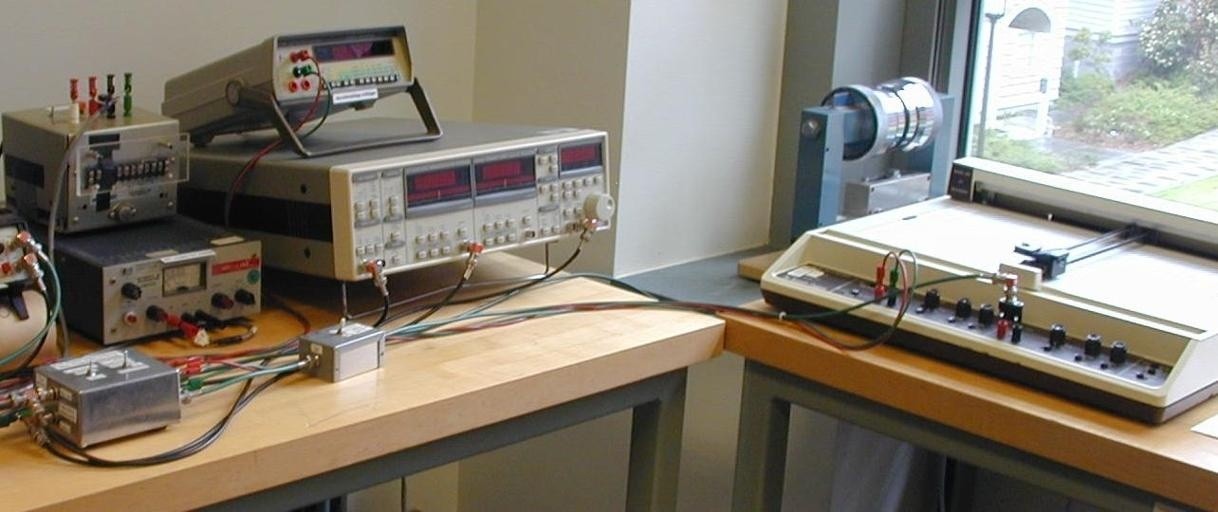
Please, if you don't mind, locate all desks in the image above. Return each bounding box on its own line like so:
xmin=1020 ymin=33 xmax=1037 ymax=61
xmin=720 ymin=300 xmax=1213 ymax=511
xmin=2 ymin=251 xmax=725 ymax=508
